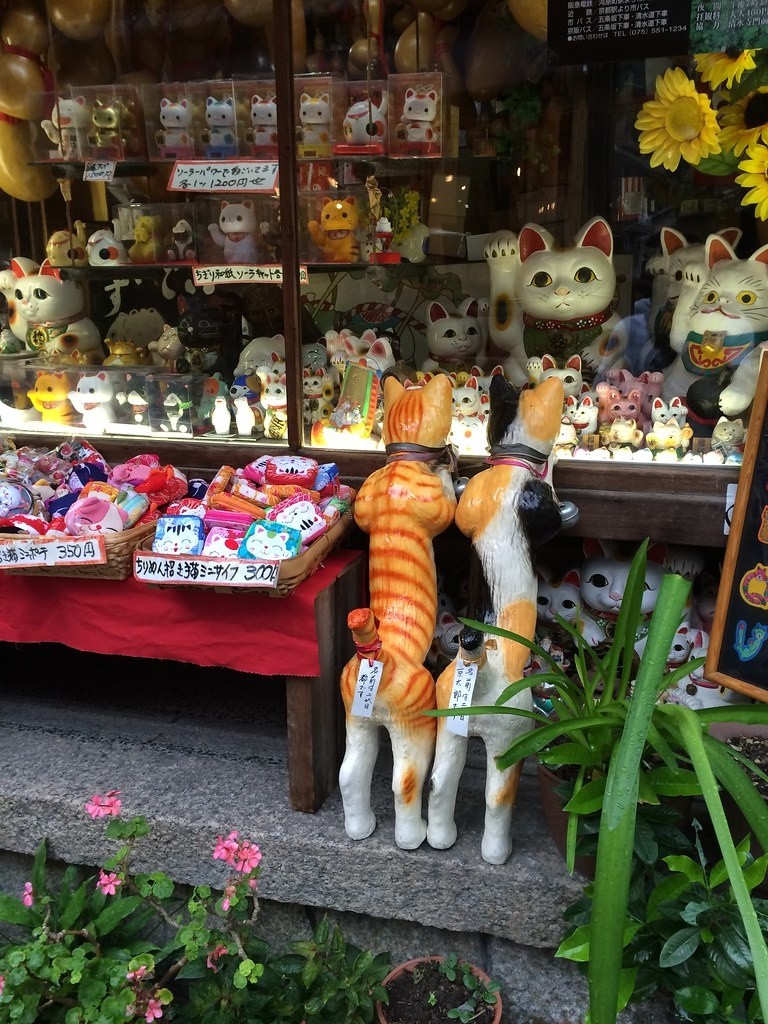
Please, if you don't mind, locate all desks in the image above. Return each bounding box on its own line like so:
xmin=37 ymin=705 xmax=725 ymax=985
xmin=0 ymin=549 xmax=368 ymax=814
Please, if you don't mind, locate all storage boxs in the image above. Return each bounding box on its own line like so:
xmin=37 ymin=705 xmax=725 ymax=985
xmin=25 ymin=70 xmax=494 ymax=265
xmin=9 ymin=355 xmax=215 ymax=438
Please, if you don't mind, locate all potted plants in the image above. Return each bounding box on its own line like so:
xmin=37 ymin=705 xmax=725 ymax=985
xmin=377 ymin=953 xmax=502 ymax=1024
xmin=419 ymin=534 xmax=768 ymax=1024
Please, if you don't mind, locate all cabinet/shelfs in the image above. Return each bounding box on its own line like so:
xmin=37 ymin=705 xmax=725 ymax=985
xmin=29 ymin=159 xmax=490 ymax=272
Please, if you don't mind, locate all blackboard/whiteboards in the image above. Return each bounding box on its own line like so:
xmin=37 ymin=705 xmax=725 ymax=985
xmin=702 ymin=350 xmax=768 ymax=705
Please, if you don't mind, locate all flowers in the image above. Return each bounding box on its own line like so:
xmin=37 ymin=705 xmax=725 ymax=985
xmin=635 ymin=47 xmax=768 ymax=221
xmin=0 ymin=785 xmax=393 ymax=1024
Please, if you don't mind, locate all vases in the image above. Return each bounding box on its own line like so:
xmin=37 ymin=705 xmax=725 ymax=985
xmin=703 ymin=721 xmax=768 ymax=900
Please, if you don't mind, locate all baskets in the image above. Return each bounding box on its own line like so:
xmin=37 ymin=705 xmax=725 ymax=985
xmin=136 ymin=497 xmax=355 ymax=595
xmin=0 ymin=516 xmax=159 ymax=580
